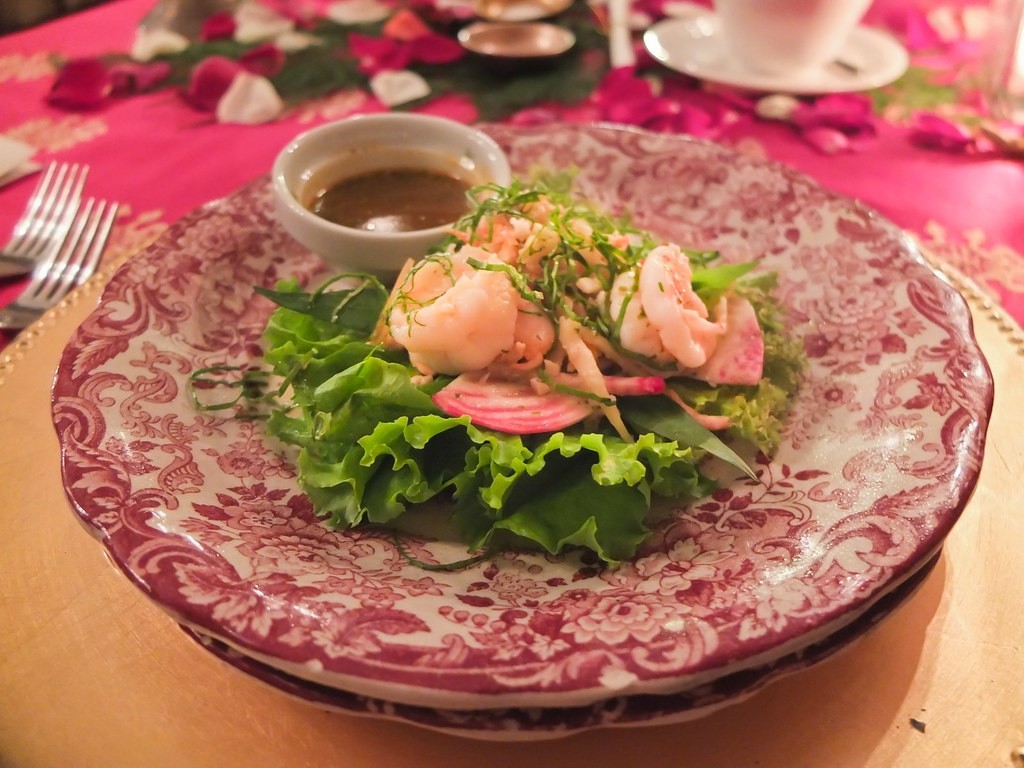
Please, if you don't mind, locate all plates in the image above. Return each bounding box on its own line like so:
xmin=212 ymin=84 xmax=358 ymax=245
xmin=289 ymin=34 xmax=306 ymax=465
xmin=475 ymin=0 xmax=572 ymax=22
xmin=643 ymin=11 xmax=909 ymax=94
xmin=52 ymin=122 xmax=994 ymax=707
xmin=457 ymin=23 xmax=576 ymax=62
xmin=177 ymin=546 xmax=942 ymax=744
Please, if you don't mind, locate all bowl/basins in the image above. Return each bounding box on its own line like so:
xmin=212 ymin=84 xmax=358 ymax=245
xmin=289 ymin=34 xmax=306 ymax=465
xmin=270 ymin=110 xmax=511 ymax=271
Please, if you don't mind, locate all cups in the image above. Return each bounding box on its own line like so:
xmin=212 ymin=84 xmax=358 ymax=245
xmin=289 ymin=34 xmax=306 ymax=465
xmin=713 ymin=0 xmax=874 ymax=78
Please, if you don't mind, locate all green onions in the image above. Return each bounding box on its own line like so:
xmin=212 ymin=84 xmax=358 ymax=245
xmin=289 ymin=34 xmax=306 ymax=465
xmin=188 ymin=168 xmax=677 ymax=411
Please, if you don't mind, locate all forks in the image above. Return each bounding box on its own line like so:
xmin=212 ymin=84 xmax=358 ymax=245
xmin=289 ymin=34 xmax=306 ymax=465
xmin=0 ymin=161 xmax=89 ymax=279
xmin=0 ymin=196 xmax=118 ymax=330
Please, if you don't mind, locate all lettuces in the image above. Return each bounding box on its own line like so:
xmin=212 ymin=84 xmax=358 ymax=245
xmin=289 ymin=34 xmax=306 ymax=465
xmin=265 ymin=250 xmax=807 ymax=566
